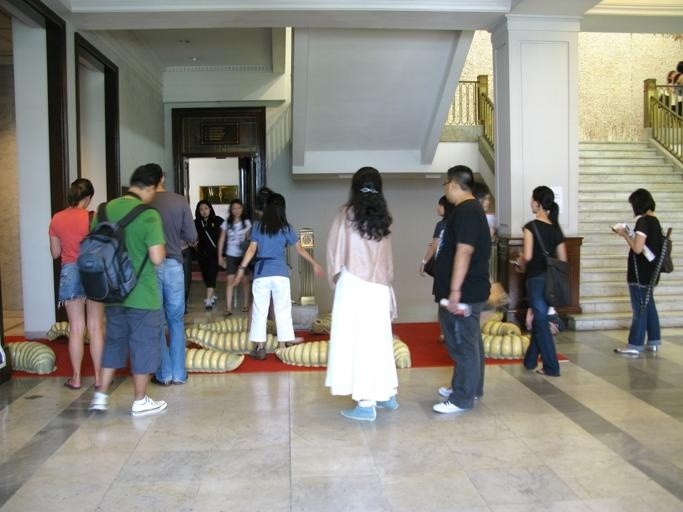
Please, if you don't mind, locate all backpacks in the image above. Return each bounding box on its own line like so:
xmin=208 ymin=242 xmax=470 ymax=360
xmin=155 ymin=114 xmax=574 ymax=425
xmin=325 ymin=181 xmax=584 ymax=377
xmin=75 ymin=202 xmax=160 ymax=303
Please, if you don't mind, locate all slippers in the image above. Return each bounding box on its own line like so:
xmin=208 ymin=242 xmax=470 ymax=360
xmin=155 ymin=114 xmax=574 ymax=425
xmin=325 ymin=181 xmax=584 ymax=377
xmin=536 ymin=368 xmax=560 ymax=377
xmin=64 ymin=379 xmax=81 ymax=389
xmin=93 ymin=380 xmax=113 ymax=389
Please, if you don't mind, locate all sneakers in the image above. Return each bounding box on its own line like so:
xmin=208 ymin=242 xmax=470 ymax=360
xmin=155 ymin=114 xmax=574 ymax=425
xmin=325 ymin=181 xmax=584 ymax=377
xmin=433 ymin=401 xmax=470 ymax=413
xmin=204 ymin=295 xmax=249 ymax=317
xmin=151 ymin=376 xmax=172 ymax=386
xmin=173 ymin=373 xmax=188 ymax=385
xmin=87 ymin=392 xmax=108 ymax=411
xmin=614 ymin=346 xmax=640 ymax=355
xmin=643 ymin=344 xmax=657 ymax=352
xmin=340 ymin=405 xmax=376 ymax=422
xmin=438 ymin=386 xmax=479 ymax=401
xmin=131 ymin=395 xmax=167 ymax=416
xmin=375 ymin=395 xmax=400 ymax=410
xmin=250 ymin=347 xmax=266 ymax=360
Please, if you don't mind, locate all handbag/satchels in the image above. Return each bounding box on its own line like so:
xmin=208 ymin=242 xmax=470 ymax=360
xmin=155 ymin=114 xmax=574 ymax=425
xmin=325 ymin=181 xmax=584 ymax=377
xmin=545 ymin=257 xmax=572 ymax=307
xmin=526 ymin=307 xmax=565 ymax=334
xmin=424 ymin=254 xmax=435 ymax=276
xmin=661 ymin=236 xmax=673 ymax=273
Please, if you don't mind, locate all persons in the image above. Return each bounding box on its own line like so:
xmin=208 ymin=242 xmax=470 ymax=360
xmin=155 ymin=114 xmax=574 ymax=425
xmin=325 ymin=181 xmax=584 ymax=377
xmin=325 ymin=167 xmax=400 ymax=420
xmin=230 ymin=191 xmax=325 ymax=350
xmin=431 ymin=165 xmax=492 ymax=414
xmin=512 ymin=186 xmax=567 ymax=377
xmin=662 ymin=59 xmax=682 ymax=118
xmin=180 ymin=185 xmax=276 ymax=321
xmin=146 ymin=170 xmax=199 ymax=384
xmin=48 ymin=177 xmax=114 ymax=389
xmin=85 ymin=165 xmax=168 ymax=417
xmin=419 ymin=183 xmax=498 ymax=343
xmin=612 ymin=188 xmax=664 ymax=355
